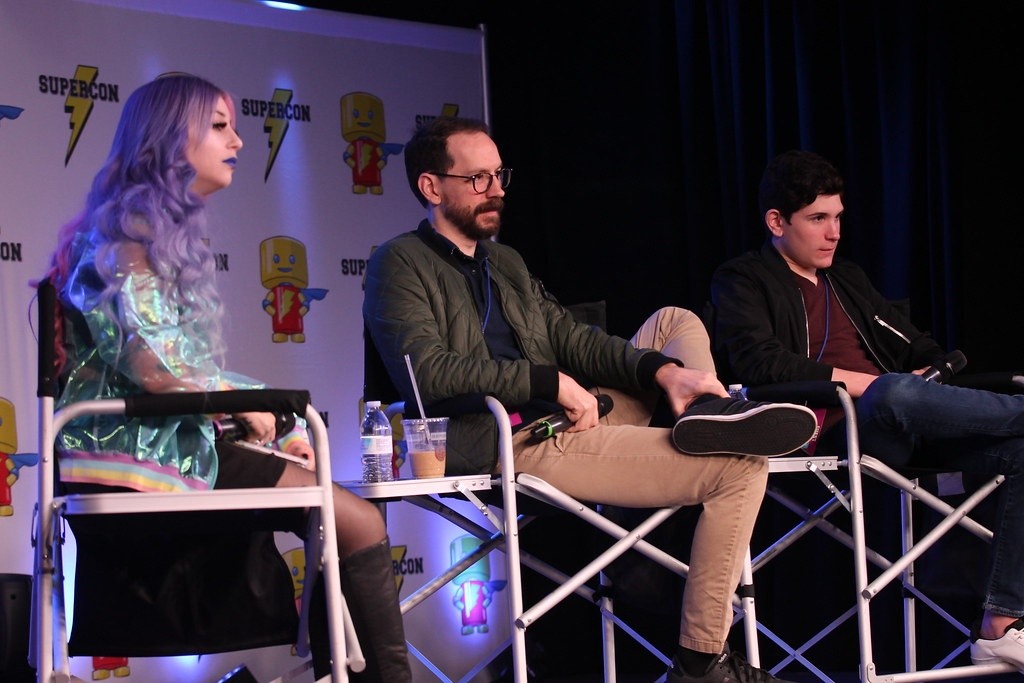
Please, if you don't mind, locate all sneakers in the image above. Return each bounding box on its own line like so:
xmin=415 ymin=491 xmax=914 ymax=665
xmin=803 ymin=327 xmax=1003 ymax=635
xmin=969 ymin=619 xmax=1024 ymax=674
xmin=671 ymin=397 xmax=818 ymax=457
xmin=666 ymin=640 xmax=791 ymax=683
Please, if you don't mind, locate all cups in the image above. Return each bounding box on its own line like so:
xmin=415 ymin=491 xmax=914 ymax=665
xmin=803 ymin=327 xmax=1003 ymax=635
xmin=400 ymin=417 xmax=449 ymax=479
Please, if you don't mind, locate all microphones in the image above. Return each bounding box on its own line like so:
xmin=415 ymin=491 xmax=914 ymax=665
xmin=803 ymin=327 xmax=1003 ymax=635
xmin=529 ymin=393 xmax=614 ymax=441
xmin=921 ymin=350 xmax=968 ymax=384
xmin=212 ymin=411 xmax=296 ymax=442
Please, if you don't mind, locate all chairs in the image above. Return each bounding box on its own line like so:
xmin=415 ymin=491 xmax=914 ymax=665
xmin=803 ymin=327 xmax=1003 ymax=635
xmin=724 ymin=368 xmax=1022 ymax=683
xmin=30 ymin=280 xmax=367 ymax=683
xmin=364 ymin=333 xmax=763 ymax=683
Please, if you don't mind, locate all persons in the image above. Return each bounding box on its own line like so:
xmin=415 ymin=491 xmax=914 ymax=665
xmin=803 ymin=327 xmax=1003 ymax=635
xmin=364 ymin=118 xmax=818 ymax=683
xmin=36 ymin=74 xmax=412 ymax=683
xmin=704 ymin=150 xmax=1023 ymax=674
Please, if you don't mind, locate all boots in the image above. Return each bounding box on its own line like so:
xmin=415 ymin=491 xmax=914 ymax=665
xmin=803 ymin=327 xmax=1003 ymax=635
xmin=308 ymin=535 xmax=412 ymax=683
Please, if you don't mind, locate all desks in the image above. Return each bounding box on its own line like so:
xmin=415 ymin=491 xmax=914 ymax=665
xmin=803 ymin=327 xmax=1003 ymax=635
xmin=339 ymin=473 xmax=502 ymax=503
xmin=762 ymin=455 xmax=849 ymax=473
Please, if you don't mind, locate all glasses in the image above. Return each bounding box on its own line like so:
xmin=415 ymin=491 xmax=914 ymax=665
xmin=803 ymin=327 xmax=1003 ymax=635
xmin=428 ymin=168 xmax=513 ymax=194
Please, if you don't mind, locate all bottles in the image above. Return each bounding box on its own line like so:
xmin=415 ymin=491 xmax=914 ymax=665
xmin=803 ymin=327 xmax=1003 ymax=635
xmin=359 ymin=401 xmax=393 ymax=483
